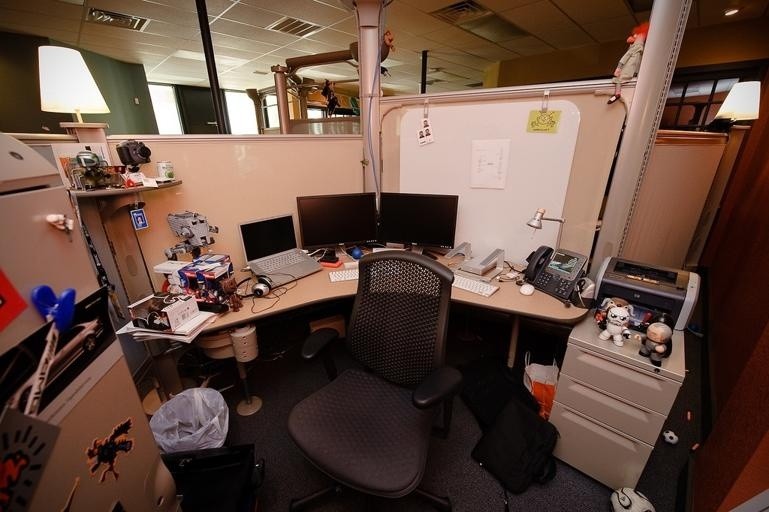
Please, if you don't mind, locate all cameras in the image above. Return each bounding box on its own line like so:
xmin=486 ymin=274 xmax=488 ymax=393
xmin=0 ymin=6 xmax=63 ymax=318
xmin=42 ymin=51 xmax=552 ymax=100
xmin=116 ymin=141 xmax=151 ymax=167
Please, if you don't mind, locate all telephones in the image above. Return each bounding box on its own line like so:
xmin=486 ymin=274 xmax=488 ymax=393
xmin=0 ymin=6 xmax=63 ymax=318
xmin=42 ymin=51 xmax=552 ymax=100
xmin=526 ymin=246 xmax=588 ymax=300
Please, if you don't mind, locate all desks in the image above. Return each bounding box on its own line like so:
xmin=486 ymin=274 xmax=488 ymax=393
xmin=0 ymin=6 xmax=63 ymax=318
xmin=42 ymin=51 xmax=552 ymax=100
xmin=173 ymin=237 xmax=596 ymax=417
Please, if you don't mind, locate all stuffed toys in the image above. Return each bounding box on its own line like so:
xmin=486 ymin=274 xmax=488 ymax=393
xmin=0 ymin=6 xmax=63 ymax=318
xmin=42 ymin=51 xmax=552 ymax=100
xmin=608 ymin=20 xmax=649 ymax=104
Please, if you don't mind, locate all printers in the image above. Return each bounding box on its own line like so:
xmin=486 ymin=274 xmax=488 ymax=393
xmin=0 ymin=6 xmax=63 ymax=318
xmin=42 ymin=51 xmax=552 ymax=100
xmin=594 ymin=256 xmax=701 ymax=334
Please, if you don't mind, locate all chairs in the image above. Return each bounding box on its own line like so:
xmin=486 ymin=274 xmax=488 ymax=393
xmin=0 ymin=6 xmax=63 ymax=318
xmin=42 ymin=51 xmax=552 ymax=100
xmin=282 ymin=250 xmax=460 ymax=512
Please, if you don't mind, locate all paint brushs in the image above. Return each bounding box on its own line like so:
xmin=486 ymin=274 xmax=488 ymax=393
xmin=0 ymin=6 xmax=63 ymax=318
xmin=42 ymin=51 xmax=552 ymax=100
xmin=535 ymin=287 xmax=570 ymax=308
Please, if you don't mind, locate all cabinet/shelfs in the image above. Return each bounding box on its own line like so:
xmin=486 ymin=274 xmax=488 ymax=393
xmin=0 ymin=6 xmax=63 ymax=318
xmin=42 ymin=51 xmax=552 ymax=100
xmin=547 ymin=308 xmax=686 ymax=492
xmin=0 ymin=132 xmax=178 ymax=512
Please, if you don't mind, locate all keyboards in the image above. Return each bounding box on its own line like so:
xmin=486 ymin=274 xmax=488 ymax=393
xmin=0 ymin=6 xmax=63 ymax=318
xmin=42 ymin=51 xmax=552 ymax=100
xmin=328 ymin=269 xmax=359 ymax=282
xmin=452 ymin=273 xmax=500 ymax=299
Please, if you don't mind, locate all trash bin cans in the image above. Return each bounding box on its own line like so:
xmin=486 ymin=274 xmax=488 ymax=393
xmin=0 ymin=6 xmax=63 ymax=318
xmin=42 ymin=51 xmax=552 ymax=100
xmin=148 ymin=387 xmax=232 ymax=454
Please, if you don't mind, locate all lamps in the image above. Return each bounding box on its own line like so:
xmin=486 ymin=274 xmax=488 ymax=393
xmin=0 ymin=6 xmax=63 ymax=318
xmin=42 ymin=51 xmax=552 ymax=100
xmin=714 ymin=81 xmax=763 ymax=122
xmin=36 ymin=44 xmax=111 ymax=128
xmin=526 ymin=208 xmax=565 ymax=251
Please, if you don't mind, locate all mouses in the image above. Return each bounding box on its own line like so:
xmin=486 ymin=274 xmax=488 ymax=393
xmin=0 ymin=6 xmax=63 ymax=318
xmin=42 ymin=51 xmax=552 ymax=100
xmin=520 ymin=283 xmax=535 ymax=296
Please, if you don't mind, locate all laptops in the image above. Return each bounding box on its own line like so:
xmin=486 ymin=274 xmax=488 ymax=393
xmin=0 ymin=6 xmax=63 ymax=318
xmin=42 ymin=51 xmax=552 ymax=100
xmin=239 ymin=214 xmax=323 ymax=290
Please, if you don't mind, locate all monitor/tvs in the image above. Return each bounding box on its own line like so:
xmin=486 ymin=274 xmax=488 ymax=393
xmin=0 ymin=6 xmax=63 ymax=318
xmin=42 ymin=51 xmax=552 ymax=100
xmin=297 ymin=192 xmax=378 ymax=264
xmin=379 ymin=192 xmax=459 ymax=255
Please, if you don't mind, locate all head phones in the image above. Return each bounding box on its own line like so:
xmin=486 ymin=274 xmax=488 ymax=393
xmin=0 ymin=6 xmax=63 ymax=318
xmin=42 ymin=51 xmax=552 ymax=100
xmin=236 ymin=274 xmax=273 ymax=300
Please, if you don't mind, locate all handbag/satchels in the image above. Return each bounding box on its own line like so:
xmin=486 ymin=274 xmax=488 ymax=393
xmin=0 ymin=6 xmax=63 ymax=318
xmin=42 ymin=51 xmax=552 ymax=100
xmin=521 ymin=352 xmax=559 ymax=423
xmin=470 ymin=396 xmax=558 ymax=495
xmin=165 ymin=443 xmax=264 ymax=512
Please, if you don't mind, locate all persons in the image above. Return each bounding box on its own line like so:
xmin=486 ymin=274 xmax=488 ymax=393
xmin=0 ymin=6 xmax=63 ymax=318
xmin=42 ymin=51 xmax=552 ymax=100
xmin=594 ymin=298 xmax=673 ymax=373
